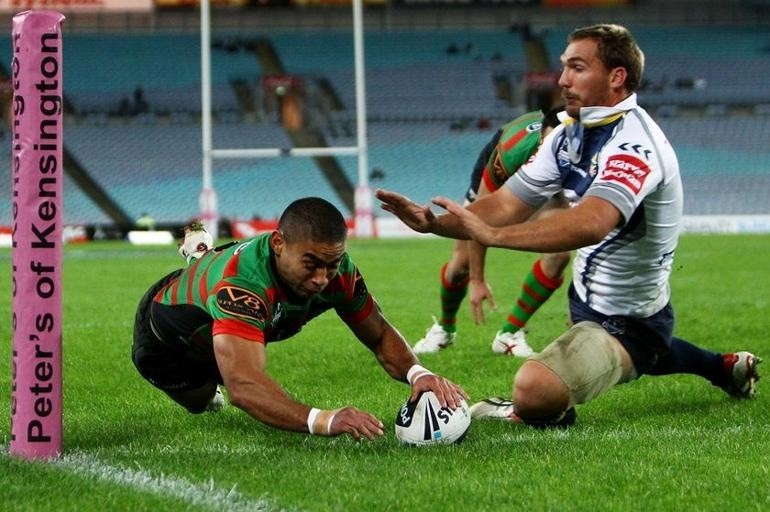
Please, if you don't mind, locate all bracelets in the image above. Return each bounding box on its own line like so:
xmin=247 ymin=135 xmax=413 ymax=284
xmin=305 ymin=404 xmax=335 ymax=437
xmin=405 ymin=364 xmax=439 ymax=383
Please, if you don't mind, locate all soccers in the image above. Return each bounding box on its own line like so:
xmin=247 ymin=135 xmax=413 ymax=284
xmin=395 ymin=389 xmax=473 ymax=449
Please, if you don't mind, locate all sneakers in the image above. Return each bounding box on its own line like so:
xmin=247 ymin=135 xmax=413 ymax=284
xmin=413 ymin=323 xmax=458 ymax=355
xmin=716 ymin=349 xmax=763 ymax=401
xmin=467 ymin=396 xmax=577 ymax=431
xmin=491 ymin=328 xmax=540 ymax=360
xmin=176 ymin=224 xmax=213 ymax=261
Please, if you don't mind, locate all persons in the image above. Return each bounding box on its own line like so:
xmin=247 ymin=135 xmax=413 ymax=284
xmin=1 ymin=73 xmax=13 ymax=123
xmin=235 ymin=65 xmax=304 ymax=131
xmin=371 ymin=22 xmax=764 ymax=428
xmin=131 ymin=196 xmax=471 ymax=442
xmin=119 ymin=88 xmax=148 ymax=117
xmin=412 ymin=103 xmax=581 ymax=358
